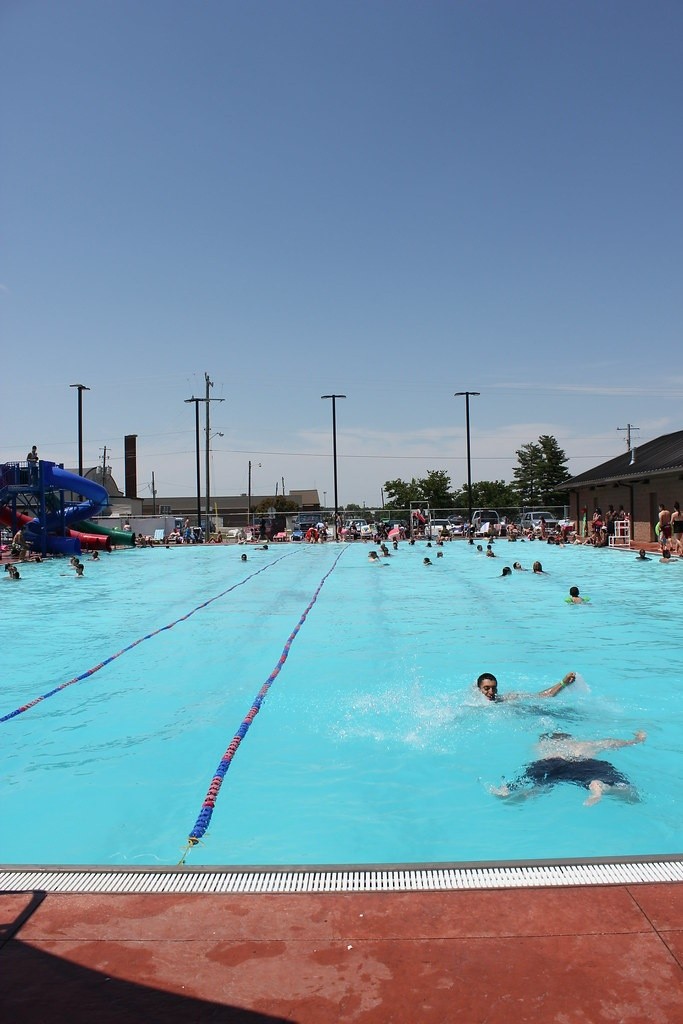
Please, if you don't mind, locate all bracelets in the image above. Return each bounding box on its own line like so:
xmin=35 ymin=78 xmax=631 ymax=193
xmin=560 ymin=681 xmax=567 ymax=688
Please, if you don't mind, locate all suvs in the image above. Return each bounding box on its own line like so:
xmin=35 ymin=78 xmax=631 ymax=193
xmin=293 ymin=513 xmax=327 ymax=536
xmin=522 ymin=512 xmax=559 ymax=533
xmin=246 ymin=516 xmax=274 ymax=541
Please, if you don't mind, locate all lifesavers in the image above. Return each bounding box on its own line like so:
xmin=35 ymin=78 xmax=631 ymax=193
xmin=566 ymin=597 xmax=590 ymax=603
xmin=655 ymin=521 xmax=674 ymax=537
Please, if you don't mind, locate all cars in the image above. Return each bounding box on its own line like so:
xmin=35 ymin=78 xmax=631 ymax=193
xmin=209 ymin=521 xmax=216 ymax=533
xmin=346 ymin=518 xmax=409 ymax=539
xmin=424 ymin=519 xmax=463 ymax=538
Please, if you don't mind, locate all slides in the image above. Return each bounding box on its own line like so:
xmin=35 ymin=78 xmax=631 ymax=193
xmin=20 ymin=460 xmax=110 ymax=557
xmin=0 ymin=504 xmax=111 ymax=550
xmin=34 ymin=492 xmax=136 ymax=546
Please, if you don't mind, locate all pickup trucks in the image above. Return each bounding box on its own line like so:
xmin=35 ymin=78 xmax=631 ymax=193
xmin=472 ymin=511 xmax=502 ymax=529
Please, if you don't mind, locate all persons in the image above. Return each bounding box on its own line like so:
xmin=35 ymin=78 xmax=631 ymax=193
xmin=73 ymin=558 xmax=79 ymax=566
xmin=237 ymin=529 xmax=247 ymax=544
xmin=35 ymin=557 xmax=42 ymax=563
xmin=76 ymin=564 xmax=84 ymax=576
xmin=255 ymin=515 xmax=269 ymax=543
xmin=184 ymin=527 xmax=191 ymax=544
xmin=5 ymin=563 xmax=20 ymax=579
xmin=123 ymin=521 xmax=131 ymax=547
xmin=249 ymin=528 xmax=259 ymax=542
xmin=255 ymin=545 xmax=269 ymax=550
xmin=168 ymin=528 xmax=180 ymax=539
xmin=136 ymin=533 xmax=147 ymax=548
xmin=91 ymin=551 xmax=100 ymax=561
xmin=635 ymin=501 xmax=683 ymax=563
xmin=305 ymin=504 xmax=512 ymax=577
xmin=206 ymin=531 xmax=222 ymax=543
xmin=506 ymin=504 xmax=625 ymax=547
xmin=185 ymin=516 xmax=190 ymax=529
xmin=513 ymin=561 xmax=591 ymax=603
xmin=241 ymin=554 xmax=246 ymax=561
xmin=477 ymin=671 xmax=576 ymax=703
xmin=70 ymin=557 xmax=75 ymax=564
xmin=27 ymin=446 xmax=38 ymax=487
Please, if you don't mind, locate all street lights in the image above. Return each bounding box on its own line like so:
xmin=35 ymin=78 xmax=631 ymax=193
xmin=184 ymin=396 xmax=210 ymax=526
xmin=70 ymin=384 xmax=91 ymax=504
xmin=321 ymin=394 xmax=347 ymax=541
xmin=203 ymin=371 xmax=225 ymax=544
xmin=453 ymin=392 xmax=480 ymax=526
